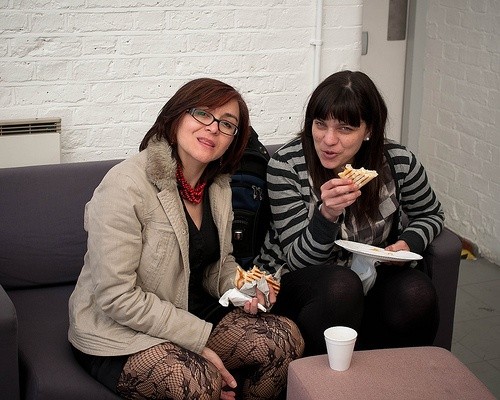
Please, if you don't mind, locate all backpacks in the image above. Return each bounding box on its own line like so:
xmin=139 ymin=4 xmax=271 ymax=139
xmin=202 ymin=126 xmax=269 ymax=272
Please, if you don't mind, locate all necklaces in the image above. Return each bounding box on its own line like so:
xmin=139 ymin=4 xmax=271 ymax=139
xmin=175 ymin=163 xmax=206 ymax=205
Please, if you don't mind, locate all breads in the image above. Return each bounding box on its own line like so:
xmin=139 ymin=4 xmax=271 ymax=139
xmin=338 ymin=164 xmax=378 ymax=189
xmin=233 ymin=264 xmax=280 ymax=295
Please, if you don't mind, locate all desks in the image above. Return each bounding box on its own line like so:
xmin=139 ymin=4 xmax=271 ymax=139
xmin=286 ymin=346 xmax=498 ymax=400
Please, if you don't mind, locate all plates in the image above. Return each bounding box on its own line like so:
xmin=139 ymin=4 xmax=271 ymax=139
xmin=334 ymin=240 xmax=424 ymax=262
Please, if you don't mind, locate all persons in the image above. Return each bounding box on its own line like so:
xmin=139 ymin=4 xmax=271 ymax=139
xmin=254 ymin=70 xmax=445 ymax=356
xmin=67 ymin=78 xmax=304 ymax=400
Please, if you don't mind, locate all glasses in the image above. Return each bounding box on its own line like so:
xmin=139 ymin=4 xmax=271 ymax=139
xmin=186 ymin=108 xmax=239 ymax=136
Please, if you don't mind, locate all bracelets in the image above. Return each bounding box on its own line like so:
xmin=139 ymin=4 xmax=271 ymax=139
xmin=318 ymin=203 xmax=339 ymax=224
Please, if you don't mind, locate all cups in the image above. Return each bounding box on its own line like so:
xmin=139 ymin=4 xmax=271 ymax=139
xmin=324 ymin=325 xmax=358 ymax=372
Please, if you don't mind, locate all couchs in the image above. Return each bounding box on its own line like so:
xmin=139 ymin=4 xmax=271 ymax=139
xmin=0 ymin=144 xmax=461 ymax=400
